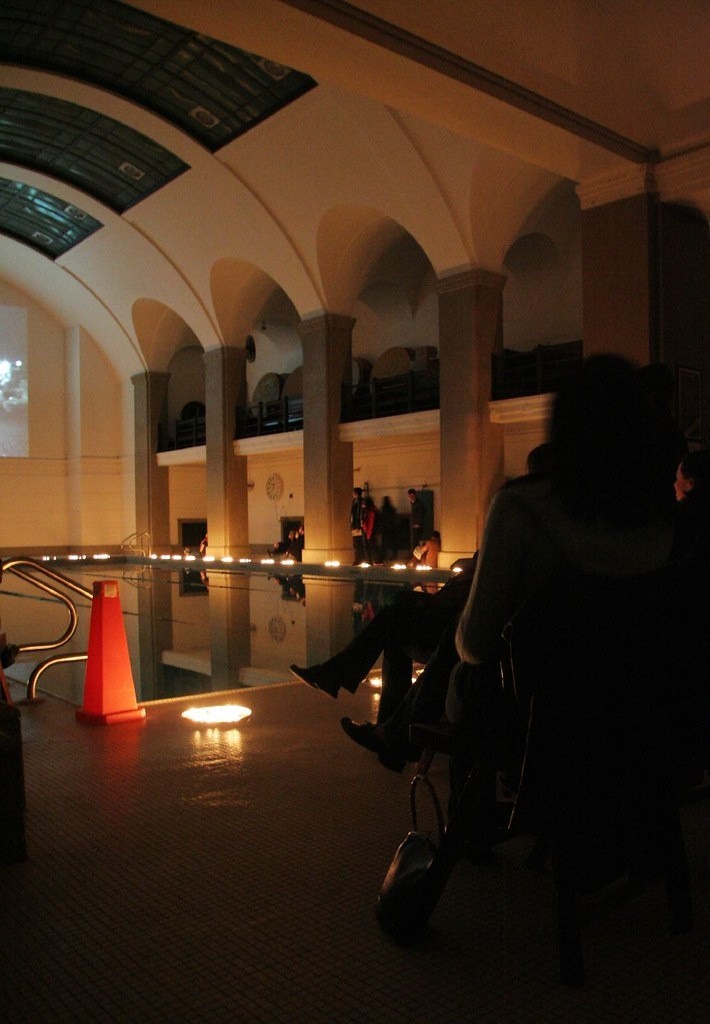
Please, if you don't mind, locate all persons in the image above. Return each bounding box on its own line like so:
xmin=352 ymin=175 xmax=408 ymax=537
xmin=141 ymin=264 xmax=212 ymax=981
xmin=288 ymin=548 xmax=480 ymax=774
xmin=199 ymin=533 xmax=209 ymax=555
xmin=672 ymin=449 xmax=710 ymax=628
xmin=338 ymin=347 xmax=691 ymax=867
xmin=274 ymin=487 xmax=440 ymax=572
xmin=634 ymin=360 xmax=691 ymax=475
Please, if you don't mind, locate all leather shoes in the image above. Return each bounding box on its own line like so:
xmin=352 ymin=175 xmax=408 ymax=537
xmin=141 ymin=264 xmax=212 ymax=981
xmin=339 ymin=715 xmax=407 ymax=774
xmin=289 ymin=663 xmax=339 ymax=699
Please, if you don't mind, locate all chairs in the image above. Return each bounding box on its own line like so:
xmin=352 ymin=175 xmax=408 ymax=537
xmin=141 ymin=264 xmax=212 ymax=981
xmin=409 ymin=575 xmax=697 ymax=988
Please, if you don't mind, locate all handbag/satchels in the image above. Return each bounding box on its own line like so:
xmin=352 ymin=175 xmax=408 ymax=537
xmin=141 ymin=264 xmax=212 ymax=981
xmin=375 ymin=777 xmax=462 ymax=963
